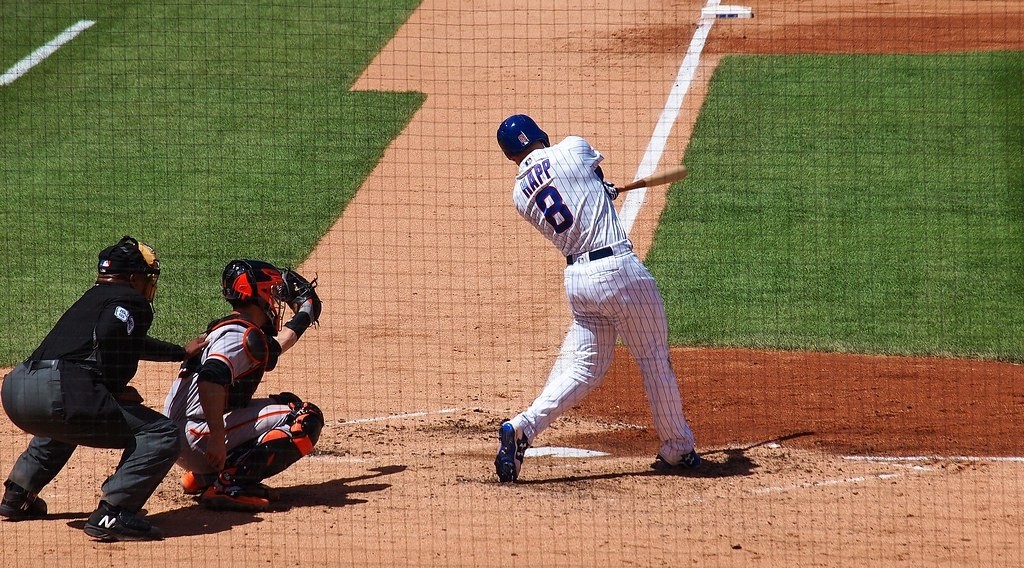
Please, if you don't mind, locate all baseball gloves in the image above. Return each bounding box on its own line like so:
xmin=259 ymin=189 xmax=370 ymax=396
xmin=276 ymin=266 xmax=322 ymax=322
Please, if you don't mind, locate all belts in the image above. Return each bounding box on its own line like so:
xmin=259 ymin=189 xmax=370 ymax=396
xmin=567 ymin=247 xmax=613 ymax=264
xmin=23 ymin=358 xmax=73 ymax=369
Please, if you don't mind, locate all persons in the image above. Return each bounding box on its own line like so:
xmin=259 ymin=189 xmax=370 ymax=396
xmin=160 ymin=258 xmax=324 ymax=513
xmin=493 ymin=115 xmax=723 ymax=483
xmin=0 ymin=235 xmax=210 ymax=542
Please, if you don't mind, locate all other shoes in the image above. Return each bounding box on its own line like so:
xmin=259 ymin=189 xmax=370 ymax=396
xmin=180 ymin=471 xmax=213 ymax=493
xmin=200 ymin=478 xmax=270 ymax=509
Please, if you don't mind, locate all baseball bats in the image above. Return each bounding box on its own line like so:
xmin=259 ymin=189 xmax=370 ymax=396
xmin=614 ymin=165 xmax=688 ymax=193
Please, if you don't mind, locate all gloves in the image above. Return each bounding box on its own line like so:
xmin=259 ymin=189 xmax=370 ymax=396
xmin=604 ymin=182 xmax=619 ymax=200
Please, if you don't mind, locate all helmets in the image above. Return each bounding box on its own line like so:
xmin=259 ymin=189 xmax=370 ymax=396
xmin=223 ymin=259 xmax=288 ymax=337
xmin=497 ymin=114 xmax=550 ymax=155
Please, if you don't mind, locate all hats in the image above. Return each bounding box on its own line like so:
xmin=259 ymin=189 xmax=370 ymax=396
xmin=97 ymin=243 xmax=154 ymax=275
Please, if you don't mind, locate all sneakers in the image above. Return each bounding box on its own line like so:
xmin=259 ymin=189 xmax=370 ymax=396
xmin=0 ymin=487 xmax=47 ymax=516
xmin=84 ymin=501 xmax=161 ymax=540
xmin=657 ymin=449 xmax=702 ymax=469
xmin=495 ymin=421 xmax=528 ymax=480
